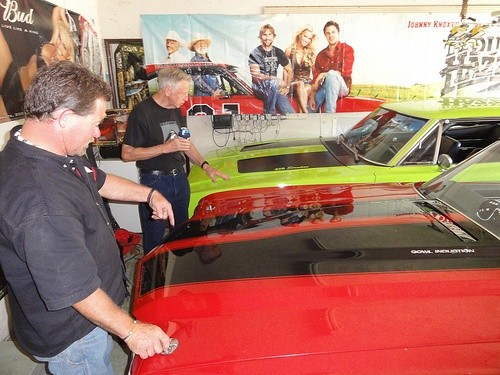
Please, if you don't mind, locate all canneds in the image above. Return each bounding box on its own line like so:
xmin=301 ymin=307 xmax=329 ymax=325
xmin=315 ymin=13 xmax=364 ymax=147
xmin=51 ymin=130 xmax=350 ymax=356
xmin=179 ymin=126 xmax=189 ymax=134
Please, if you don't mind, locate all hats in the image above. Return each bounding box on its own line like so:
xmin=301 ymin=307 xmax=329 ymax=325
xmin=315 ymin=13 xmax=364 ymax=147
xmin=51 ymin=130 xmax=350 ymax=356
xmin=186 ymin=32 xmax=211 ymax=51
xmin=158 ymin=30 xmax=184 ymax=46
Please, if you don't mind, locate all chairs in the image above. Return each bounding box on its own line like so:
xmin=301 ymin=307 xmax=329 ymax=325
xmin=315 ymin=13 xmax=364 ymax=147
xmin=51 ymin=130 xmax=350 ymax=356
xmin=441 ymin=135 xmax=461 ymax=164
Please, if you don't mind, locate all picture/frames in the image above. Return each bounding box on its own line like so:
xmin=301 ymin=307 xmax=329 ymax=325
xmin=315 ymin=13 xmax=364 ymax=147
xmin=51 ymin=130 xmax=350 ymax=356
xmin=104 ymin=38 xmax=149 ymax=113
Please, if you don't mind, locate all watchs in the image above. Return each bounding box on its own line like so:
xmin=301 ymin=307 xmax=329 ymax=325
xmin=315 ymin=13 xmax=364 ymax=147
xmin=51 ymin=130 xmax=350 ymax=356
xmin=201 ymin=161 xmax=209 ymax=169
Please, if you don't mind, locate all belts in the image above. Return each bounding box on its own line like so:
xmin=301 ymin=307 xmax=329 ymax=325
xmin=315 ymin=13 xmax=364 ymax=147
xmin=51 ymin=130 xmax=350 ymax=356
xmin=142 ymin=168 xmax=183 ymax=176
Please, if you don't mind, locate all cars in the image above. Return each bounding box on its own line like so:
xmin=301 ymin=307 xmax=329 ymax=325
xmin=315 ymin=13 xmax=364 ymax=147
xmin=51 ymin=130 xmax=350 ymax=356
xmin=119 ymin=140 xmax=500 ymax=375
xmin=94 ymin=63 xmax=388 ymax=159
xmin=184 ymin=93 xmax=500 ymax=182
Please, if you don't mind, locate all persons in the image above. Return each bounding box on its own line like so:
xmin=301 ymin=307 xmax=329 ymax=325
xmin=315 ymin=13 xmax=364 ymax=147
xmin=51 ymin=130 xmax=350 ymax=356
xmin=158 ymin=30 xmax=187 ymax=72
xmin=0 ymin=60 xmax=174 ymax=375
xmin=188 ymin=33 xmax=220 ymax=96
xmin=283 ymin=24 xmax=317 ymax=114
xmin=248 ymin=24 xmax=293 ymax=114
xmin=306 ymin=21 xmax=354 ymax=113
xmin=122 ymin=67 xmax=230 ymax=256
xmin=0 ymin=7 xmax=74 ymax=123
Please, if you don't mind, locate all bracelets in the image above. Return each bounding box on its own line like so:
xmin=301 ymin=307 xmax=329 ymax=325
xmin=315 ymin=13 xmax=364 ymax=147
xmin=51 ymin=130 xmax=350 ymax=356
xmin=147 ymin=188 xmax=156 ymax=203
xmin=122 ymin=320 xmax=137 ymax=340
xmin=283 ymin=85 xmax=290 ymax=88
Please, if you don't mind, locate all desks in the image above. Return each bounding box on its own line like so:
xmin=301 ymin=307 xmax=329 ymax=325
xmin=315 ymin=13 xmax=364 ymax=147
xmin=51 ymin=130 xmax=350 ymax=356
xmin=96 ymin=156 xmax=143 ymax=233
